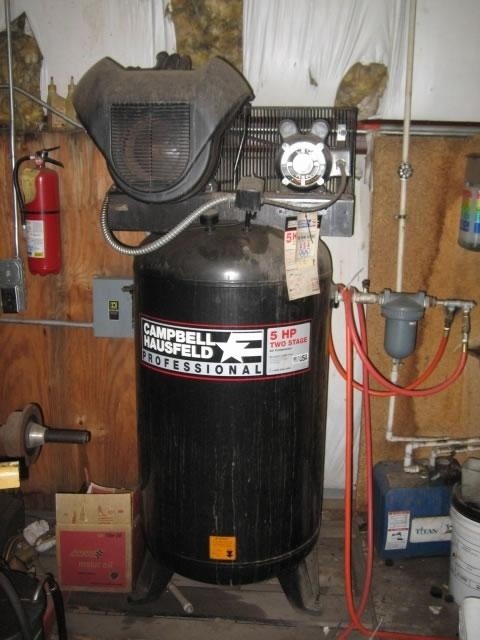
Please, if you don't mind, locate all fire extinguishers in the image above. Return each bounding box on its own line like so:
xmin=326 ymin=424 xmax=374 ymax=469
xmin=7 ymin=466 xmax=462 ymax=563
xmin=13 ymin=145 xmax=64 ymax=276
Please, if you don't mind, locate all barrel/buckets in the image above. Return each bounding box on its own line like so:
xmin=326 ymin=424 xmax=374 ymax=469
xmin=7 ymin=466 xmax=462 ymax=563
xmin=449 ymin=482 xmax=479 ymax=605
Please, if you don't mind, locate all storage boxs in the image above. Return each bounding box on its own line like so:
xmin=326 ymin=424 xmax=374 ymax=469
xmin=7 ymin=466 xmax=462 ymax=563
xmin=54 ymin=482 xmax=143 ymax=594
